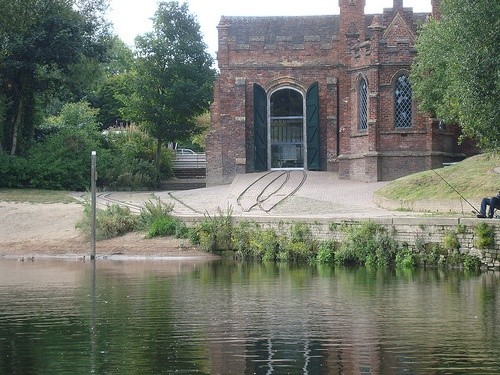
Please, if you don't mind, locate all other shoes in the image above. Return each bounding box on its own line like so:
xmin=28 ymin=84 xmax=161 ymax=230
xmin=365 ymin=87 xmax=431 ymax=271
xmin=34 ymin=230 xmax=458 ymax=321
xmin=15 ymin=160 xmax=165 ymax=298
xmin=488 ymin=214 xmax=493 ymax=218
xmin=477 ymin=213 xmax=486 ymax=217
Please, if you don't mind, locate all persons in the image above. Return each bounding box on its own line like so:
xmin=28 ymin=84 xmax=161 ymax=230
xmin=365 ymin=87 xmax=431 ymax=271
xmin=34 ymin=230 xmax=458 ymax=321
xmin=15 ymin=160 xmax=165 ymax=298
xmin=477 ymin=190 xmax=500 ymax=218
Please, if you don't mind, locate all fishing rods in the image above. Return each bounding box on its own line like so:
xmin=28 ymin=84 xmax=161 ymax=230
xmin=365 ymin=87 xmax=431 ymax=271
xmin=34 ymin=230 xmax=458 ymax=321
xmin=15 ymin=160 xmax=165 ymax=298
xmin=415 ymin=153 xmax=481 ymax=215
